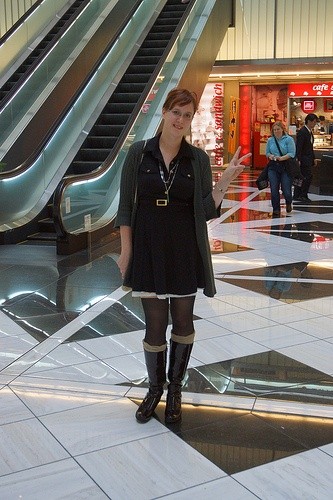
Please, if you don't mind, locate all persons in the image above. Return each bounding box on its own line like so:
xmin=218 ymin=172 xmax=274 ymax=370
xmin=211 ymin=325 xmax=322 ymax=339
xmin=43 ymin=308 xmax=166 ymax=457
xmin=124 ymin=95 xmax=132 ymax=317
xmin=293 ymin=113 xmax=318 ymax=202
xmin=320 ymin=115 xmax=333 ymax=134
xmin=117 ymin=88 xmax=252 ymax=424
xmin=266 ymin=121 xmax=296 ymax=217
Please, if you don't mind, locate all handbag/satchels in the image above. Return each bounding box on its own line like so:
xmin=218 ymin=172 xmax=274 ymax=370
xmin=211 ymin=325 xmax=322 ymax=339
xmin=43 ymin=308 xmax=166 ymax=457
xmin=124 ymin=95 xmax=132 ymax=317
xmin=286 ymin=158 xmax=302 ymax=178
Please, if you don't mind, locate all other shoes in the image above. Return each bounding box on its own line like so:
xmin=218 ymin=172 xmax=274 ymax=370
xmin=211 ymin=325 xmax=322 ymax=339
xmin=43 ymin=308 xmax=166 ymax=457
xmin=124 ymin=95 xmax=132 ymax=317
xmin=272 ymin=210 xmax=279 ymax=218
xmin=294 ymin=193 xmax=312 ymax=202
xmin=286 ymin=204 xmax=292 ymax=212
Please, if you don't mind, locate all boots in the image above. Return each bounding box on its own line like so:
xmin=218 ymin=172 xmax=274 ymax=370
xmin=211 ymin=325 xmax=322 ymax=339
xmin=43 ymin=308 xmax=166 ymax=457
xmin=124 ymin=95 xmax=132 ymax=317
xmin=164 ymin=330 xmax=196 ymax=424
xmin=136 ymin=338 xmax=168 ymax=423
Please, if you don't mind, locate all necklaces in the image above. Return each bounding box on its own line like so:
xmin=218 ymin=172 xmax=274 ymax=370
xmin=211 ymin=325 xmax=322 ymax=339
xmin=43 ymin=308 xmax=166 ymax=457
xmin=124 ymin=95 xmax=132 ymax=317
xmin=158 ymin=161 xmax=181 ymax=203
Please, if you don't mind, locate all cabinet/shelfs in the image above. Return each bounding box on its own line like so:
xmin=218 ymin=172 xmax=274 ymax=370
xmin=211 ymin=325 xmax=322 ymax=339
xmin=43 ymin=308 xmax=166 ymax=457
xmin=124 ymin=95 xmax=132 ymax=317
xmin=254 ymin=121 xmax=274 ymax=169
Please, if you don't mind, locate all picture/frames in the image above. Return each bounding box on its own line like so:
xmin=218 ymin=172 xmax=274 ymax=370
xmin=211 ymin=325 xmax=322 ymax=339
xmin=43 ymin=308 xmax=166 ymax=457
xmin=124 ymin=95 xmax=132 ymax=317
xmin=324 ymin=98 xmax=333 ymax=112
xmin=304 ymin=101 xmax=314 ymax=110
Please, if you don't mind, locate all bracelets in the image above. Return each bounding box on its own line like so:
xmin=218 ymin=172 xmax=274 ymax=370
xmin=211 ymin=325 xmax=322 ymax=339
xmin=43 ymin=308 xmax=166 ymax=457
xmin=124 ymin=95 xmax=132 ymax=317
xmin=214 ymin=182 xmax=228 ymax=192
